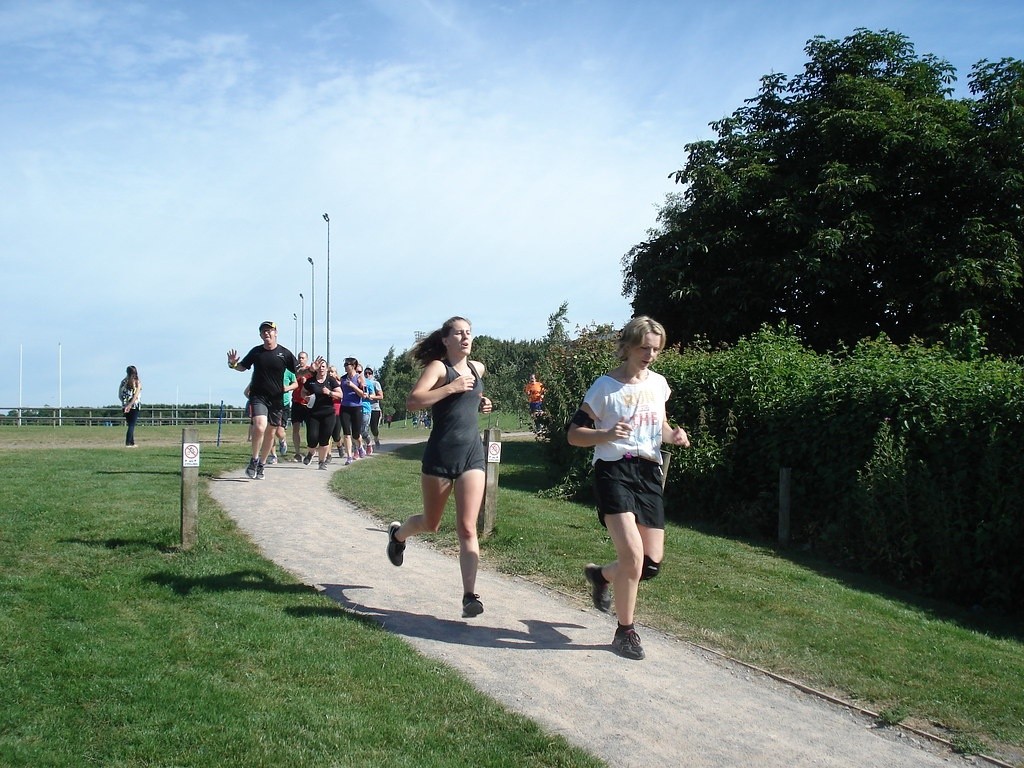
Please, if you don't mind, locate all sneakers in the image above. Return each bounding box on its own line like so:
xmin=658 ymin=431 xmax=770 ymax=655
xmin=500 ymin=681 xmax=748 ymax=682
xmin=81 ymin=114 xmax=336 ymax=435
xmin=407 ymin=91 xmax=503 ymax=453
xmin=256 ymin=463 xmax=265 ymax=479
xmin=584 ymin=561 xmax=611 ymax=612
xmin=612 ymin=627 xmax=645 ymax=659
xmin=462 ymin=593 xmax=484 ymax=618
xmin=353 ymin=449 xmax=359 ymax=460
xmin=386 ymin=521 xmax=407 ymax=566
xmin=366 ymin=443 xmax=373 ymax=455
xmin=303 ymin=451 xmax=317 ymax=465
xmin=345 ymin=457 xmax=351 ymax=465
xmin=293 ymin=455 xmax=302 ymax=462
xmin=318 ymin=461 xmax=327 ymax=469
xmin=268 ymin=455 xmax=278 ymax=464
xmin=279 ymin=440 xmax=287 ymax=455
xmin=359 ymin=447 xmax=365 ymax=458
xmin=246 ymin=459 xmax=258 ymax=478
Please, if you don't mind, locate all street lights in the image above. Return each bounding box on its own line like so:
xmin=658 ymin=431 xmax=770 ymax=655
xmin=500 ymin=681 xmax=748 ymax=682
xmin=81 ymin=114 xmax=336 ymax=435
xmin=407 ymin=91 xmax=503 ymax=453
xmin=293 ymin=313 xmax=297 ymax=358
xmin=300 ymin=293 xmax=304 ymax=352
xmin=308 ymin=256 xmax=315 ymax=362
xmin=321 ymin=212 xmax=332 ymax=366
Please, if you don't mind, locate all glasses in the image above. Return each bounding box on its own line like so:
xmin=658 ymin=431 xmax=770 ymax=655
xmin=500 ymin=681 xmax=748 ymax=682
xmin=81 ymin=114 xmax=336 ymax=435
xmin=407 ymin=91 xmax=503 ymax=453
xmin=364 ymin=372 xmax=373 ymax=375
xmin=356 ymin=370 xmax=362 ymax=374
xmin=344 ymin=363 xmax=354 ymax=367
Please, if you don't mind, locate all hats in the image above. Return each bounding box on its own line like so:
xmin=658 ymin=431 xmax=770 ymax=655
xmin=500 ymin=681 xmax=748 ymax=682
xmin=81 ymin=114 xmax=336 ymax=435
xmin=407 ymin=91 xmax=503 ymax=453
xmin=259 ymin=321 xmax=276 ymax=331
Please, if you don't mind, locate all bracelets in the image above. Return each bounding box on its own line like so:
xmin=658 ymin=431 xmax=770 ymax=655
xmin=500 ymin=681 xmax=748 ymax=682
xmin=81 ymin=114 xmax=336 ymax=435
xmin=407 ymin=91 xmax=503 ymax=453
xmin=228 ymin=361 xmax=238 ymax=369
xmin=329 ymin=390 xmax=334 ymax=396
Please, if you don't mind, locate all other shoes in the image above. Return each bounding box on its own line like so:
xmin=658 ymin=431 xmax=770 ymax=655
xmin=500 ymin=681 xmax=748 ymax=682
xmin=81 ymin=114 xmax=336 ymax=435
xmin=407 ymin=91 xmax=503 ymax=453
xmin=125 ymin=444 xmax=138 ymax=448
xmin=374 ymin=440 xmax=380 ymax=449
xmin=325 ymin=455 xmax=332 ymax=463
xmin=337 ymin=444 xmax=345 ymax=458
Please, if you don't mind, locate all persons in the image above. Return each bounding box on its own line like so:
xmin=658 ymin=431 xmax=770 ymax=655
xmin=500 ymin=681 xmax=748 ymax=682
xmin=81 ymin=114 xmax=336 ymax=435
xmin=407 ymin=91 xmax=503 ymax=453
xmin=525 ymin=373 xmax=549 ymax=430
xmin=301 ymin=359 xmax=343 ymax=470
xmin=387 ymin=316 xmax=493 ymax=618
xmin=355 ymin=364 xmax=375 ymax=455
xmin=341 ymin=357 xmax=367 ymax=466
xmin=268 ymin=368 xmax=299 ymax=465
xmin=227 ymin=320 xmax=325 ymax=479
xmin=327 ymin=366 xmax=344 ymax=463
xmin=566 ymin=314 xmax=691 ymax=660
xmin=365 ymin=366 xmax=384 ymax=449
xmin=118 ymin=365 xmax=142 ymax=450
xmin=291 ymin=352 xmax=313 ymax=463
xmin=386 ymin=412 xmax=432 ymax=429
